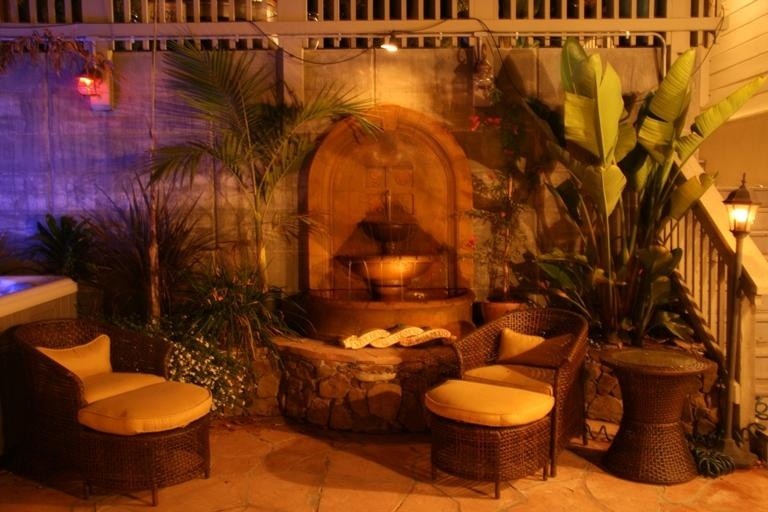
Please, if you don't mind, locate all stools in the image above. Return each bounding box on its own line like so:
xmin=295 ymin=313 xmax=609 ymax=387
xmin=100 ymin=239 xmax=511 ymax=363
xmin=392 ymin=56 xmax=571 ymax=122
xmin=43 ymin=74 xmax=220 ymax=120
xmin=424 ymin=378 xmax=557 ymax=498
xmin=77 ymin=382 xmax=211 ymax=505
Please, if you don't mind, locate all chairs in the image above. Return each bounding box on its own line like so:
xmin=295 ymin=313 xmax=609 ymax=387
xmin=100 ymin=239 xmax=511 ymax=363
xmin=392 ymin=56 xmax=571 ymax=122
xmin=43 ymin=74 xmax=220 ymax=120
xmin=9 ymin=318 xmax=173 ymax=496
xmin=456 ymin=307 xmax=590 ymax=476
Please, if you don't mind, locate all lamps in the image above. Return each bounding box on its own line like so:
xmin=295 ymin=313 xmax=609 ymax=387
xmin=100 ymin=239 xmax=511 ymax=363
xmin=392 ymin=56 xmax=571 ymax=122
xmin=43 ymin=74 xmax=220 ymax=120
xmin=473 ymin=37 xmax=496 ymax=107
xmin=715 ymin=172 xmax=760 ymax=470
xmin=75 ymin=53 xmax=103 ymax=97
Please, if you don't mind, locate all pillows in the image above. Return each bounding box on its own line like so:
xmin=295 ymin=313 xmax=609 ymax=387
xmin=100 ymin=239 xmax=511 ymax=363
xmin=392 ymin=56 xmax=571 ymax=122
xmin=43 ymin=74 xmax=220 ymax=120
xmin=36 ymin=334 xmax=113 ymax=379
xmin=499 ymin=327 xmax=574 ymax=366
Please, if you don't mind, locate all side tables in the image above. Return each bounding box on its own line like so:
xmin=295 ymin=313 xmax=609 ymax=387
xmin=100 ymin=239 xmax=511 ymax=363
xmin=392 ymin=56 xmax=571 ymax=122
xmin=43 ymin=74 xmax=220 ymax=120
xmin=598 ymin=345 xmax=713 ymax=484
